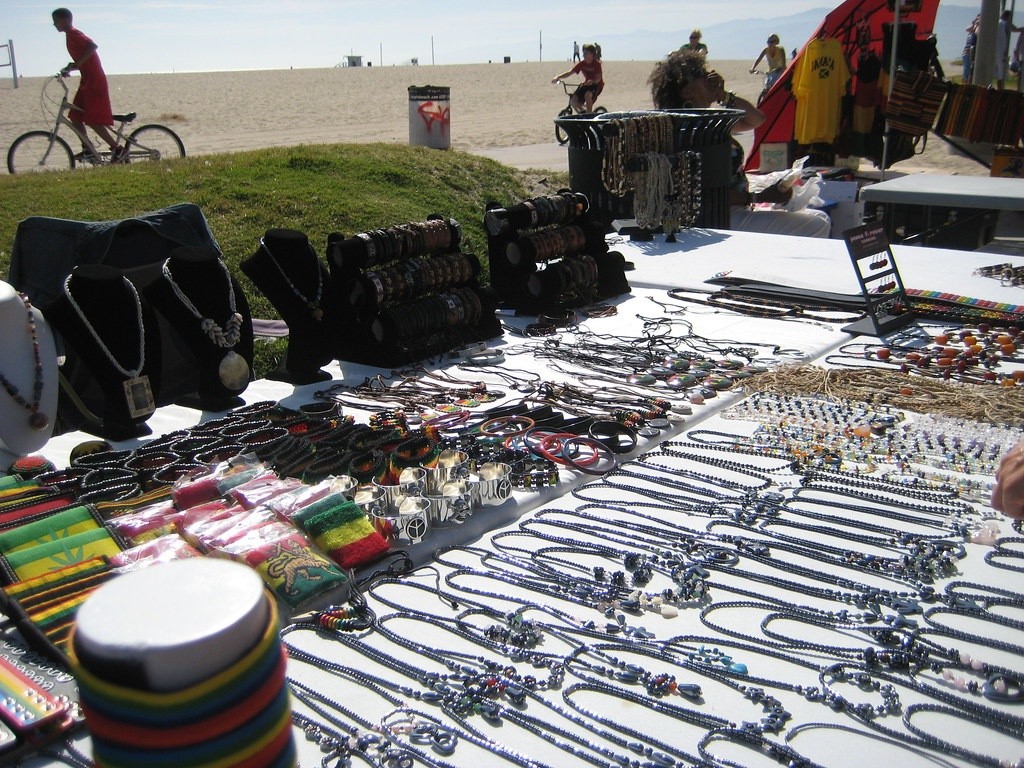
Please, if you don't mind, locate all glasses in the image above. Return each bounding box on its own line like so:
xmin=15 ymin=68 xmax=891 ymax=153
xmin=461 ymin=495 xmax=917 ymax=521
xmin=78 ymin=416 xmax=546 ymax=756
xmin=689 ymin=36 xmax=698 ymax=39
xmin=767 ymin=40 xmax=778 ymax=43
xmin=683 ymin=66 xmax=706 ymax=81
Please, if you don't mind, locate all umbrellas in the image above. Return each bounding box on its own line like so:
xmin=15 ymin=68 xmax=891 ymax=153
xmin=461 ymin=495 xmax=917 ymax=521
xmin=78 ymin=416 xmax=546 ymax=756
xmin=741 ymin=0 xmax=939 ymax=174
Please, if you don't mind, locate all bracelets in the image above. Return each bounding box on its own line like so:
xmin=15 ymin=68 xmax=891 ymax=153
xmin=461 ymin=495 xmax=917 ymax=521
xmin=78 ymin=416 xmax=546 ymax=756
xmin=717 ymin=91 xmax=736 ymax=108
xmin=422 ymin=391 xmax=637 ymax=474
xmin=357 ymin=180 xmax=614 ymax=363
xmin=37 ymin=399 xmax=559 ymax=606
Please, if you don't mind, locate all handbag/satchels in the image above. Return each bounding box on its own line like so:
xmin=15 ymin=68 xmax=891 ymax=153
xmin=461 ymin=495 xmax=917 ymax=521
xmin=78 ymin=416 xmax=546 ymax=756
xmin=884 ymin=69 xmax=947 ymax=135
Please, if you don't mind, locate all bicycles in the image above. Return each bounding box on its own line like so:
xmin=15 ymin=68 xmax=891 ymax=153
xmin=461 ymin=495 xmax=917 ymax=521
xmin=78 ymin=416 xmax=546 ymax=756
xmin=750 ymin=67 xmax=786 ymax=109
xmin=552 ymin=80 xmax=609 ymax=144
xmin=6 ymin=69 xmax=186 ymax=177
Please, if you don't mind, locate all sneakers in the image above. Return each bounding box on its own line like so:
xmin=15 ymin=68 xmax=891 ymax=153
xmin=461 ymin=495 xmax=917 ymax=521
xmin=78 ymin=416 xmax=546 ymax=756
xmin=72 ymin=143 xmax=96 ymax=160
xmin=108 ymin=144 xmax=129 ymax=163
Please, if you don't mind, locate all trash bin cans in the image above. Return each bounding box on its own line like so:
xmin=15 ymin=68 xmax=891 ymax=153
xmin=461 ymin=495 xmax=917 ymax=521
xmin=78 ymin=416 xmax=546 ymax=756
xmin=407 ymin=84 xmax=451 ymax=152
xmin=554 ymin=108 xmax=747 ymax=230
xmin=368 ymin=62 xmax=371 ymax=66
xmin=504 ymin=56 xmax=510 ymax=63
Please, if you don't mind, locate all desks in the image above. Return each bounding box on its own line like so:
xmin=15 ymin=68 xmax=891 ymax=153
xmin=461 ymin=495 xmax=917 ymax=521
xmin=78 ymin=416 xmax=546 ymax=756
xmin=858 ymin=170 xmax=1024 ymax=256
xmin=0 ymin=218 xmax=1024 ymax=768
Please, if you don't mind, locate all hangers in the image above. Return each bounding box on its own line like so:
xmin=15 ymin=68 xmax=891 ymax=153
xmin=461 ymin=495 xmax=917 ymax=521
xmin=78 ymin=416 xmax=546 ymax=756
xmin=816 ymin=24 xmax=834 ymax=42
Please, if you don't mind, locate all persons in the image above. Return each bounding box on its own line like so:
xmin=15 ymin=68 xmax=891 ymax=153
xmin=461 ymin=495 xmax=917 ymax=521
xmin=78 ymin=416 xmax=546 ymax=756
xmin=52 ymin=8 xmax=125 ymax=163
xmin=648 ymin=29 xmax=832 ymax=238
xmin=552 ymin=41 xmax=604 ymax=114
xmin=960 ymin=9 xmax=1024 ymax=90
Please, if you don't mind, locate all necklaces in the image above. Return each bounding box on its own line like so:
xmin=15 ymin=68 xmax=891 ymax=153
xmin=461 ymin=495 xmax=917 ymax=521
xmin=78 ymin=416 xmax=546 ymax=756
xmin=281 ymin=430 xmax=1024 ymax=768
xmin=39 ymin=734 xmax=94 ymax=768
xmin=601 ymin=112 xmax=703 ymax=234
xmin=314 ymin=363 xmax=506 ymax=414
xmin=536 ymin=287 xmax=867 ymax=402
xmin=723 ymin=320 xmax=1024 ymax=489
xmin=0 ymin=232 xmax=321 ymax=427
